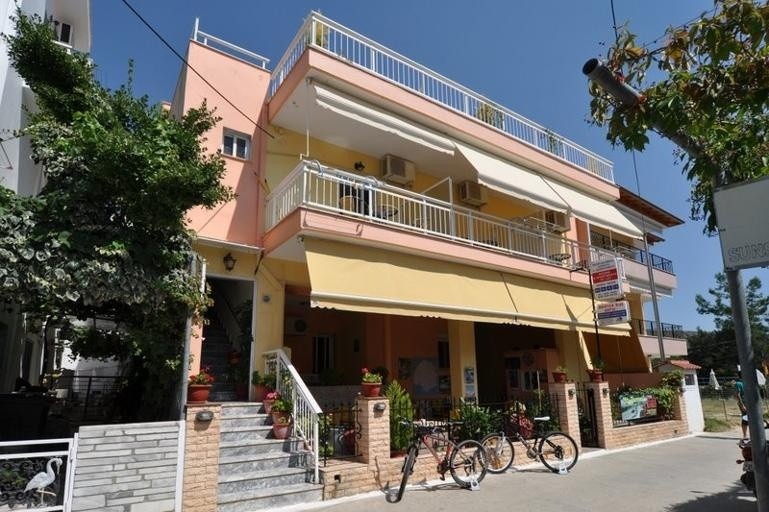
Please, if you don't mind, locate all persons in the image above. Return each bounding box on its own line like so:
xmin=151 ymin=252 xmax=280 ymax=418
xmin=735 ymin=369 xmax=749 ymax=440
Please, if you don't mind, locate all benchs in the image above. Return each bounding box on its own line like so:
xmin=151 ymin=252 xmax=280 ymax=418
xmin=339 ymin=195 xmax=373 ymax=218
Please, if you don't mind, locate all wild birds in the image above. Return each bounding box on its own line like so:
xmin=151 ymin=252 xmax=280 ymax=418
xmin=22 ymin=457 xmax=64 ymax=507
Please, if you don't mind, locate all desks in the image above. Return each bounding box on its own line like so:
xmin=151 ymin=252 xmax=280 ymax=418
xmin=367 ymin=203 xmax=399 ymax=221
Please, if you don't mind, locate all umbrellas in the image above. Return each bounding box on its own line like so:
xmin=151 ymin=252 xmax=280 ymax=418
xmin=707 ymin=367 xmax=720 ymax=391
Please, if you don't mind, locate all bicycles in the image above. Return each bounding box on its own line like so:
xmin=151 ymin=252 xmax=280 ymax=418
xmin=397 ymin=415 xmax=490 ymax=501
xmin=477 ymin=406 xmax=579 ymax=474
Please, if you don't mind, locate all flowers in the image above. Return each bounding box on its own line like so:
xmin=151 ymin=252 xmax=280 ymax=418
xmin=188 ymin=369 xmax=214 ymax=385
xmin=263 ymin=390 xmax=280 ymax=399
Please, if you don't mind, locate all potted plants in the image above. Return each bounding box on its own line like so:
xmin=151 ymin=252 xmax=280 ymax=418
xmin=228 ymin=333 xmax=254 ymax=401
xmin=268 ymin=396 xmax=294 ymax=438
xmin=551 ymin=355 xmax=609 ymax=383
xmin=251 ymin=368 xmax=277 ymax=402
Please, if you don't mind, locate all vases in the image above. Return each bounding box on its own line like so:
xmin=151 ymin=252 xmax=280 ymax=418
xmin=263 ymin=400 xmax=275 ymax=413
xmin=187 ymin=383 xmax=213 ymax=403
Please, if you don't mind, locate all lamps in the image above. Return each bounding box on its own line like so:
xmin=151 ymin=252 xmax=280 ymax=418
xmin=223 ymin=253 xmax=237 ymax=272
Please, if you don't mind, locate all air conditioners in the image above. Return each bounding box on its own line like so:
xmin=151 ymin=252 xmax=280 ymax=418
xmin=380 ymin=154 xmax=415 ymax=187
xmin=458 ymin=180 xmax=488 ymax=208
